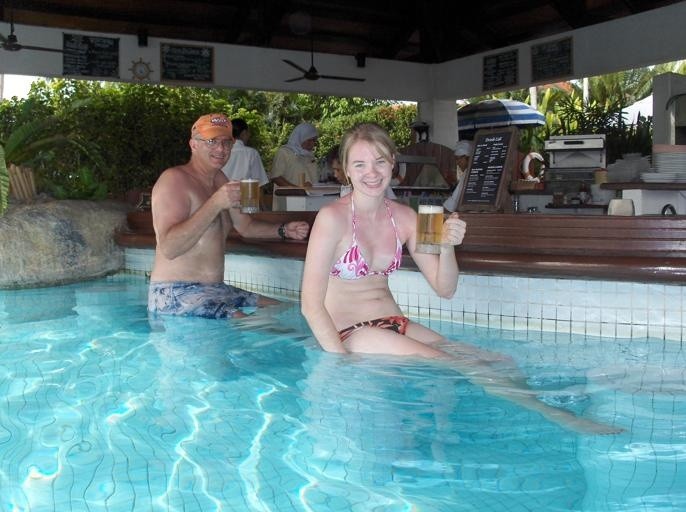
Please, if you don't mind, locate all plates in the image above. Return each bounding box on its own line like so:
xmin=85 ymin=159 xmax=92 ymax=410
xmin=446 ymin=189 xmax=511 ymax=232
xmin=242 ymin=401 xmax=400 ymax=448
xmin=606 ymin=143 xmax=686 ymax=186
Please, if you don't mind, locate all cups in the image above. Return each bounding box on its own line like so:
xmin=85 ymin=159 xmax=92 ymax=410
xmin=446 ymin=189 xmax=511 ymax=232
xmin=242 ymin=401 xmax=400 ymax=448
xmin=231 ymin=178 xmax=261 ymax=215
xmin=415 ymin=203 xmax=450 ymax=256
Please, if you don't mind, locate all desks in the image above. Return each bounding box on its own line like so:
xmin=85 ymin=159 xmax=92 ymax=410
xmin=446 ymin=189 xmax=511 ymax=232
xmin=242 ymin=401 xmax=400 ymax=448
xmin=545 ymin=204 xmax=608 ymax=215
xmin=274 ymin=186 xmax=452 ymax=212
xmin=600 ymin=182 xmax=686 ymax=216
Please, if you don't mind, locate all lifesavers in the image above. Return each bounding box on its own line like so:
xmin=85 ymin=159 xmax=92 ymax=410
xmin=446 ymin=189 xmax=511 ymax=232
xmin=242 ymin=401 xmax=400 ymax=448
xmin=523 ymin=153 xmax=544 ymax=183
xmin=390 ymin=152 xmax=406 ymax=186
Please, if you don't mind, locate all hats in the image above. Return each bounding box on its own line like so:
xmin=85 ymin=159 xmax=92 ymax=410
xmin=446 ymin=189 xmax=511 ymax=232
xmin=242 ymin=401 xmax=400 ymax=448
xmin=192 ymin=114 xmax=235 ymax=144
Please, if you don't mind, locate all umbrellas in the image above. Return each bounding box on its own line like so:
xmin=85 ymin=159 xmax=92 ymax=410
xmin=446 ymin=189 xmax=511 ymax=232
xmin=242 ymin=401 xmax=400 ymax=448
xmin=458 ymin=99 xmax=545 ymax=131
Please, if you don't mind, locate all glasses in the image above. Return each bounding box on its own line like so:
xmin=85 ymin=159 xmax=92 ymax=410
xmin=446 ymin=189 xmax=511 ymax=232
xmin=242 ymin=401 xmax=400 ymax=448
xmin=194 ymin=137 xmax=233 ymax=150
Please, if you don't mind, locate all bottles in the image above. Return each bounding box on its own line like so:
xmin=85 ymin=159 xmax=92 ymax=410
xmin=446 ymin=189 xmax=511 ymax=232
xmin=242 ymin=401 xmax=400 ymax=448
xmin=577 ymin=182 xmax=588 ymax=204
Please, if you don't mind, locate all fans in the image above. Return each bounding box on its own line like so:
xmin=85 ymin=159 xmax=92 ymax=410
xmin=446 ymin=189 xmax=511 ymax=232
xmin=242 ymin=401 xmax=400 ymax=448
xmin=0 ymin=6 xmax=65 ymax=52
xmin=281 ymin=34 xmax=366 ymax=83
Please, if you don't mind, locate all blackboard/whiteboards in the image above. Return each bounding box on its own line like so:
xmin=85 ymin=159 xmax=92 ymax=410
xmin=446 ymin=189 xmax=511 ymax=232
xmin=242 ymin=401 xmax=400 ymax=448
xmin=457 ymin=126 xmax=518 ymax=212
xmin=529 ymin=36 xmax=576 ymax=84
xmin=61 ymin=32 xmax=121 ymax=80
xmin=481 ymin=48 xmax=520 ymax=94
xmin=159 ymin=41 xmax=216 ymax=84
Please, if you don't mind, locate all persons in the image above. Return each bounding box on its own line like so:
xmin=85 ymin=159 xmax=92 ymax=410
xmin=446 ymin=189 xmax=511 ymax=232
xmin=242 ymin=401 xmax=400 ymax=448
xmin=443 ymin=140 xmax=473 ymax=214
xmin=327 ymin=144 xmax=398 ymax=200
xmin=301 ymin=121 xmax=625 ymax=436
xmin=148 ymin=114 xmax=282 ymax=320
xmin=271 ymin=123 xmax=318 ymax=212
xmin=220 ymin=119 xmax=270 ymax=213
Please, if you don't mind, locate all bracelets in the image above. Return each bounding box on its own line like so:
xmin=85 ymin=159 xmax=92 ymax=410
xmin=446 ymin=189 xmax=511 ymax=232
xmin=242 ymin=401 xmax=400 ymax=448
xmin=279 ymin=224 xmax=286 ymax=240
